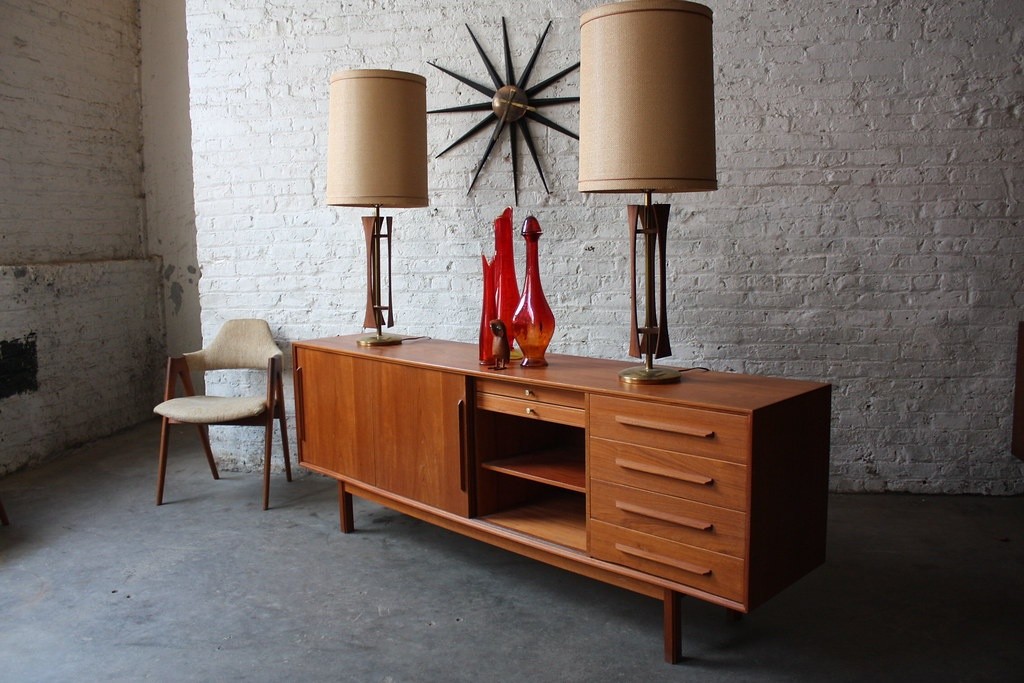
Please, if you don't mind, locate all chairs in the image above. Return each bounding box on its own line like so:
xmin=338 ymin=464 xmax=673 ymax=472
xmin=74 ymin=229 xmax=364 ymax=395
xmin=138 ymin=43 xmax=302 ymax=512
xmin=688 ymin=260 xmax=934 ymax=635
xmin=151 ymin=317 xmax=292 ymax=511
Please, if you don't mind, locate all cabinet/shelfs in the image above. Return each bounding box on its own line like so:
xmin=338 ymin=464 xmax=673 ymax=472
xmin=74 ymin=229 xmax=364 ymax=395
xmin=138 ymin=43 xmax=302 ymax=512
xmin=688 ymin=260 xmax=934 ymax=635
xmin=292 ymin=330 xmax=836 ymax=665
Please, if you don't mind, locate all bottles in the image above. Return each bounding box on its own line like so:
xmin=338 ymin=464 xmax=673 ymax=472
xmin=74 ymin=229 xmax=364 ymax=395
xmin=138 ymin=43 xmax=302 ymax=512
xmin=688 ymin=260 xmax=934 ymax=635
xmin=511 ymin=216 xmax=555 ymax=367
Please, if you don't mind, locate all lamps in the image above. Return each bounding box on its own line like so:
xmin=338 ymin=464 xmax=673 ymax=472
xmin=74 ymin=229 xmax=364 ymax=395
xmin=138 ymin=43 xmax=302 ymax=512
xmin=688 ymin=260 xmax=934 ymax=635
xmin=578 ymin=0 xmax=719 ymax=388
xmin=326 ymin=67 xmax=432 ymax=348
